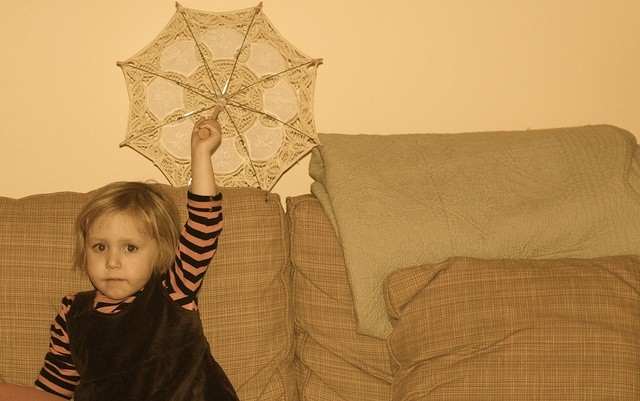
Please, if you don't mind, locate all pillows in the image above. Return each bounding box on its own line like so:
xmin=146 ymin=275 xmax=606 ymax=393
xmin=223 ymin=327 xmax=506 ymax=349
xmin=381 ymin=255 xmax=635 ymax=400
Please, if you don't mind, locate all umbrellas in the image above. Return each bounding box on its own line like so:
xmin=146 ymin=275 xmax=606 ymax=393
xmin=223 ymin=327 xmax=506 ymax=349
xmin=114 ymin=0 xmax=324 ymax=193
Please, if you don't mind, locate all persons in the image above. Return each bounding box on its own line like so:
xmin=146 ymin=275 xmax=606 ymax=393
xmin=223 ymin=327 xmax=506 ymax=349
xmin=1 ymin=116 xmax=238 ymax=401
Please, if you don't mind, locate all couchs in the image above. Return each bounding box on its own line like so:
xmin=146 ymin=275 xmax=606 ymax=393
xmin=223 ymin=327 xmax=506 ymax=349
xmin=284 ymin=195 xmax=636 ymax=399
xmin=0 ymin=184 xmax=298 ymax=400
